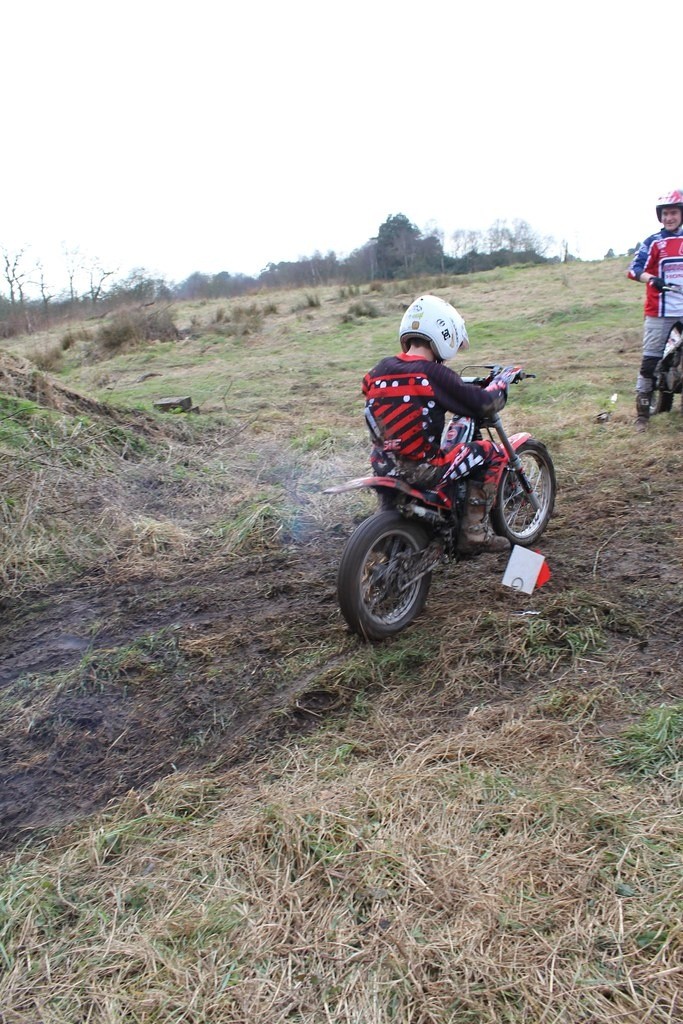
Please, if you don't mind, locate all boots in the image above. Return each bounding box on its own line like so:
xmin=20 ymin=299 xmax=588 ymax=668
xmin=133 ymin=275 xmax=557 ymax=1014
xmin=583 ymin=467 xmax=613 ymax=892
xmin=634 ymin=394 xmax=651 ymax=432
xmin=455 ymin=479 xmax=510 ymax=559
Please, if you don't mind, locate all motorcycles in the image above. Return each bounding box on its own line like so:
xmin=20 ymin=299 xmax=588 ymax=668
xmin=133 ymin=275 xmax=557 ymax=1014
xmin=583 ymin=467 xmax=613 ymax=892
xmin=324 ymin=363 xmax=556 ymax=643
xmin=649 ymin=283 xmax=683 ymax=415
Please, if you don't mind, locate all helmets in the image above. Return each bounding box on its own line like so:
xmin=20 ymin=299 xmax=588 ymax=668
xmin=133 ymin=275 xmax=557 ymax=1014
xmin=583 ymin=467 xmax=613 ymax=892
xmin=656 ymin=190 xmax=683 ymax=228
xmin=399 ymin=295 xmax=470 ymax=362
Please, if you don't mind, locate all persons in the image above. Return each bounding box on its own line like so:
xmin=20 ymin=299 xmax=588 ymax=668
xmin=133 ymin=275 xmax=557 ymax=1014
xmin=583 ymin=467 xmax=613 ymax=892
xmin=361 ymin=293 xmax=514 ymax=554
xmin=628 ymin=188 xmax=683 ymax=432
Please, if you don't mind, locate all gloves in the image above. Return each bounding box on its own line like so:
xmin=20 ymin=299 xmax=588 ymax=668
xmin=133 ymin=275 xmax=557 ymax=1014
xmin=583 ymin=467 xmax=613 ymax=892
xmin=490 ymin=365 xmax=523 ymax=388
